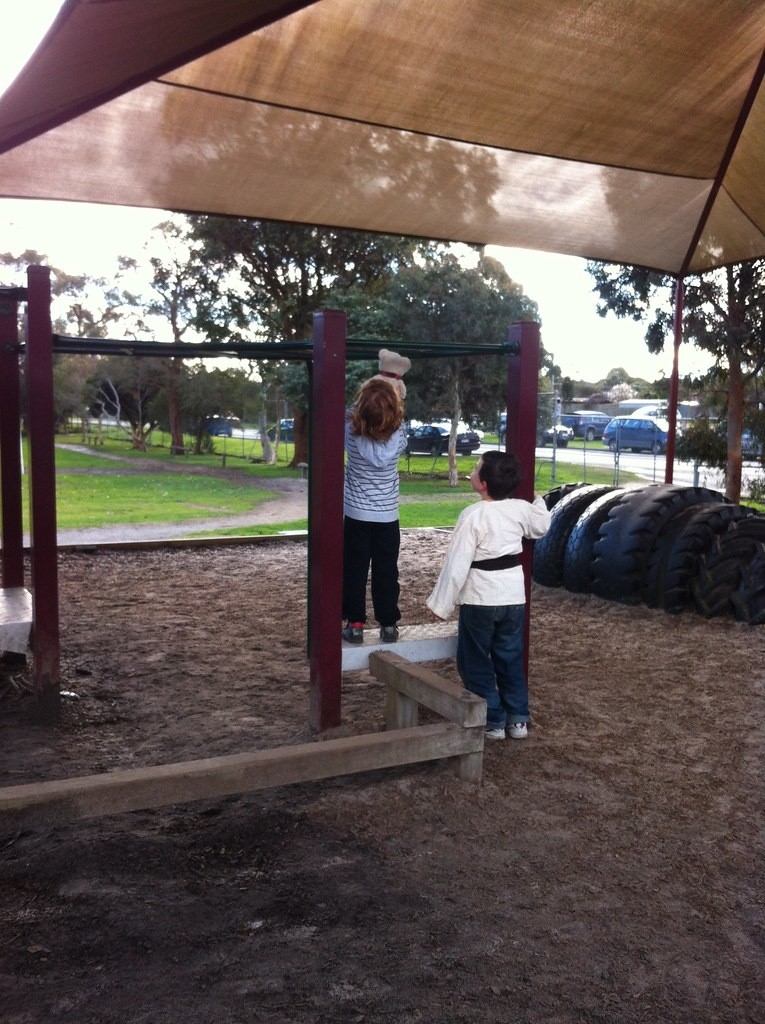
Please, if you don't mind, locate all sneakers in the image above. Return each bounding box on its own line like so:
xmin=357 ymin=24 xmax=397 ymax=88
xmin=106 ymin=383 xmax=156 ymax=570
xmin=484 ymin=728 xmax=508 ymax=745
xmin=508 ymin=721 xmax=528 ymax=739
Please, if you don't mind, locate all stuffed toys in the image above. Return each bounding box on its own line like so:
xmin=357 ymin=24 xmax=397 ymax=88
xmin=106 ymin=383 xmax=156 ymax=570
xmin=359 ymin=348 xmax=412 ymax=414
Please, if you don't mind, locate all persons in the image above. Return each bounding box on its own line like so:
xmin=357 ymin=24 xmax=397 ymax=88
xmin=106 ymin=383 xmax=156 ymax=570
xmin=426 ymin=451 xmax=551 ymax=738
xmin=344 ymin=379 xmax=409 ymax=644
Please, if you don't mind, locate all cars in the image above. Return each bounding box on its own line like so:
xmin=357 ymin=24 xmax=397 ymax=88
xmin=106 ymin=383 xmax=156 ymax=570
xmin=462 ymin=412 xmax=574 ymax=448
xmin=400 ymin=417 xmax=481 ymax=456
xmin=206 ymin=414 xmax=241 ymax=437
xmin=267 ymin=419 xmax=294 ymax=442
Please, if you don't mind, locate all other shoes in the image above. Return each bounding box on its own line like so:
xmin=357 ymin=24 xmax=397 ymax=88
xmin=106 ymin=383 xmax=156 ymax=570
xmin=342 ymin=623 xmax=363 ymax=643
xmin=379 ymin=625 xmax=399 ymax=642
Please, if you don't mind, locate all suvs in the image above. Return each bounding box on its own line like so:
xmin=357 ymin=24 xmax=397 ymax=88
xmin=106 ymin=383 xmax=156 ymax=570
xmin=712 ymin=420 xmax=765 ymax=460
xmin=631 ymin=406 xmax=682 ymax=419
xmin=601 ymin=417 xmax=684 ymax=455
xmin=562 ymin=411 xmax=613 ymax=441
